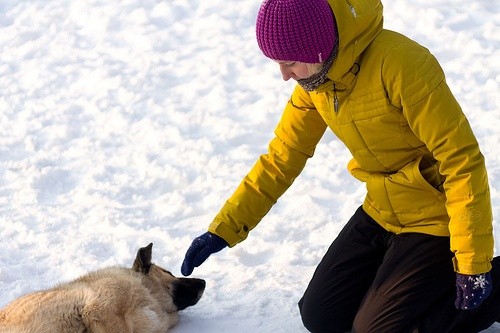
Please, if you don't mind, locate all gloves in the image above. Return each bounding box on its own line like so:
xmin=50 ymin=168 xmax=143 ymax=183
xmin=181 ymin=230 xmax=228 ymax=275
xmin=455 ymin=269 xmax=492 ymax=310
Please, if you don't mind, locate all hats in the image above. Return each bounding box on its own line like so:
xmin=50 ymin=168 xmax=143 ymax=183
xmin=255 ymin=0 xmax=334 ymax=63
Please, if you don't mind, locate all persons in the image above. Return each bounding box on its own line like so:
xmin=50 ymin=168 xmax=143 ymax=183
xmin=181 ymin=0 xmax=500 ymax=333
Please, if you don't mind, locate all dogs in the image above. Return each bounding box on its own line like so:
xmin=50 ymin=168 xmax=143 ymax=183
xmin=0 ymin=242 xmax=207 ymax=333
